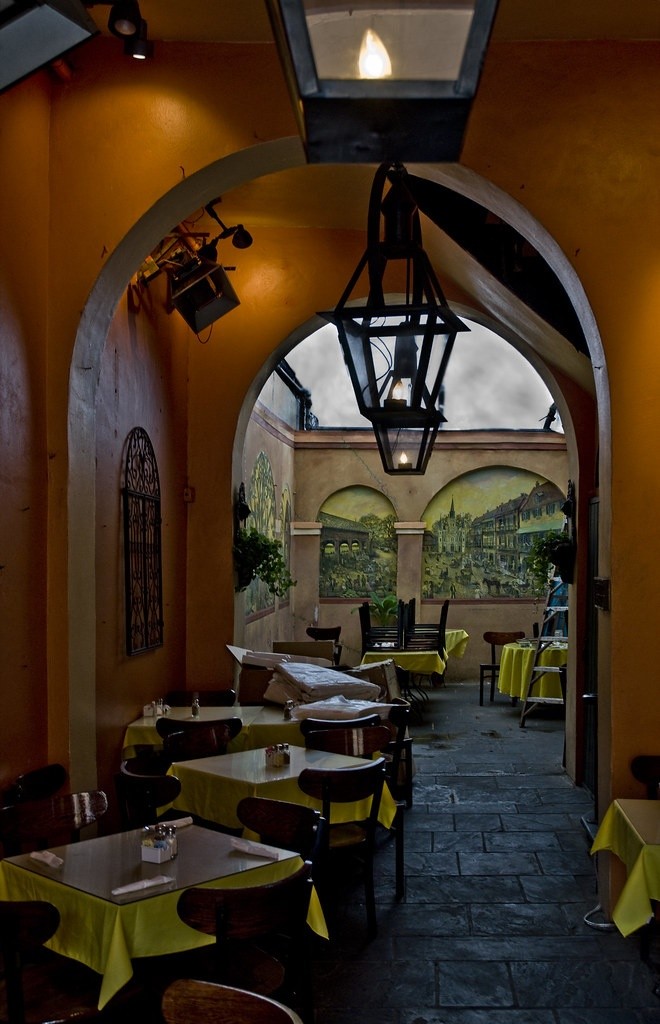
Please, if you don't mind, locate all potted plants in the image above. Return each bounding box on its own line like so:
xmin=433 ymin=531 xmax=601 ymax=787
xmin=233 ymin=526 xmax=298 ymax=598
xmin=522 ymin=529 xmax=576 ymax=614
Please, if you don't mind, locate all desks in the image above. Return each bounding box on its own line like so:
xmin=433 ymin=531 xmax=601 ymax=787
xmin=497 ymin=641 xmax=568 ymax=707
xmin=0 ymin=822 xmax=329 ymax=1024
xmin=121 ymin=705 xmax=307 ymax=759
xmin=589 ymin=799 xmax=660 ymax=938
xmin=360 ymin=629 xmax=470 ymax=722
xmin=155 ymin=743 xmax=397 ymax=838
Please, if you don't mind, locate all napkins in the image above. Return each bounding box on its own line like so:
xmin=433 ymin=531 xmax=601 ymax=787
xmin=111 ymin=874 xmax=176 ymax=896
xmin=230 ymin=836 xmax=280 ymax=860
xmin=30 ymin=848 xmax=65 ymax=869
xmin=155 ymin=816 xmax=193 ymax=828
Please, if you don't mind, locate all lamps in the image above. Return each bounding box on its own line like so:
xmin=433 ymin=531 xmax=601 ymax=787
xmin=264 ymin=0 xmax=501 ymax=475
xmin=0 ymin=0 xmax=152 ymax=93
xmin=135 ymin=196 xmax=253 ymax=343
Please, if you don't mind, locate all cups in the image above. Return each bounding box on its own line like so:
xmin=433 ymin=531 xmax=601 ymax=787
xmin=555 ymin=630 xmax=563 ymax=637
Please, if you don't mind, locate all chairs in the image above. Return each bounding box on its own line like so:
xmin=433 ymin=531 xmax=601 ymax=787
xmin=0 ymin=598 xmax=660 ymax=1024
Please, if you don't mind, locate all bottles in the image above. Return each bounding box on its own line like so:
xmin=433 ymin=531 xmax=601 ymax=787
xmin=155 ymin=824 xmax=166 ymax=841
xmin=283 ymin=743 xmax=290 ymax=765
xmin=156 ymin=699 xmax=163 ymax=715
xmin=191 ymin=699 xmax=200 ymax=718
xmin=272 ymin=744 xmax=284 ymax=766
xmin=166 ymin=825 xmax=178 ymax=858
xmin=284 ymin=700 xmax=294 ymax=719
